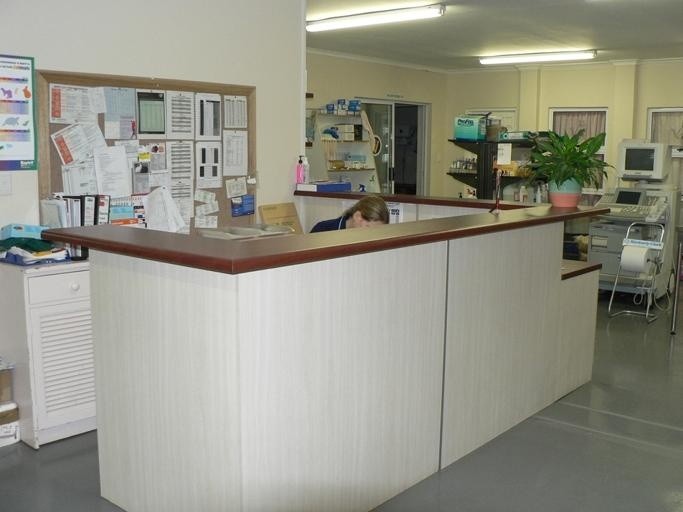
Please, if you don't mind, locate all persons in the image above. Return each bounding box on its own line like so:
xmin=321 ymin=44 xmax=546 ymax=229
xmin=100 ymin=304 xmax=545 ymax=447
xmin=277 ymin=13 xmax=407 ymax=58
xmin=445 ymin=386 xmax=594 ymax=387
xmin=309 ymin=196 xmax=389 ymax=235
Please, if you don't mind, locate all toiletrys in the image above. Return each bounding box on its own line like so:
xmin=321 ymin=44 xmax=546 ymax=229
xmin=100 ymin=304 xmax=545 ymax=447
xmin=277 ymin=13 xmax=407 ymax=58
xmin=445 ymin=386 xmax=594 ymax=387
xmin=301 ymin=157 xmax=310 ymax=183
xmin=296 ymin=155 xmax=305 ymax=183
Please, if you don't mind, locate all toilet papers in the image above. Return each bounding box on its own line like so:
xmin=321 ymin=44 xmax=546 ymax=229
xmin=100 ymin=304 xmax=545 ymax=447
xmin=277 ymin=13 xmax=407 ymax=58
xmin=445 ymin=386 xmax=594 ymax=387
xmin=621 ymin=246 xmax=652 ymax=274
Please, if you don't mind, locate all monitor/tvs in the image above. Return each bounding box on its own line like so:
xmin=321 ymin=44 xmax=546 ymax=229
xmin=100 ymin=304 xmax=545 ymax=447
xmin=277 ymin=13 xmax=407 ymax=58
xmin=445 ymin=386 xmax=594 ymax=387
xmin=620 ymin=143 xmax=671 ymax=189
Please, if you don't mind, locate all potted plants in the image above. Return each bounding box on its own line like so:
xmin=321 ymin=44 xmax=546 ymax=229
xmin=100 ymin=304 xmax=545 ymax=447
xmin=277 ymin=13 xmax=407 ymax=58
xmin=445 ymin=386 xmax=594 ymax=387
xmin=524 ymin=128 xmax=615 ymax=208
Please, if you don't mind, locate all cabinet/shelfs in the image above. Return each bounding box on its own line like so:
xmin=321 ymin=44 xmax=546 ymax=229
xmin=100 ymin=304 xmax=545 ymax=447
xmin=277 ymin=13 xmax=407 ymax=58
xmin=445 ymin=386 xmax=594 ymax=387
xmin=0 ymin=259 xmax=96 ymax=450
xmin=315 ymin=112 xmax=383 ymax=193
xmin=445 ymin=139 xmax=529 ymax=200
